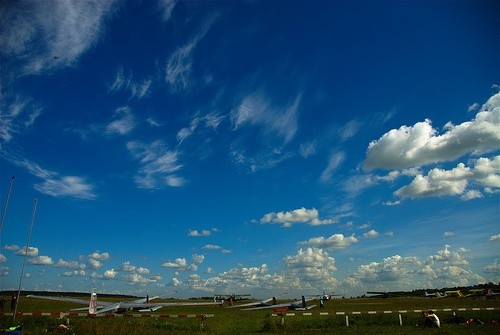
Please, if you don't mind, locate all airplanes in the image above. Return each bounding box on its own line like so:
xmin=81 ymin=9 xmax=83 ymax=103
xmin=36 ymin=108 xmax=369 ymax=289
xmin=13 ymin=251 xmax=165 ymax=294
xmin=226 ymin=292 xmax=288 ymax=307
xmin=25 ymin=294 xmax=225 ymax=314
xmin=240 ymin=296 xmax=318 ymax=311
xmin=303 ymin=290 xmax=364 ymax=300
xmin=425 ymin=289 xmax=499 ymax=301
